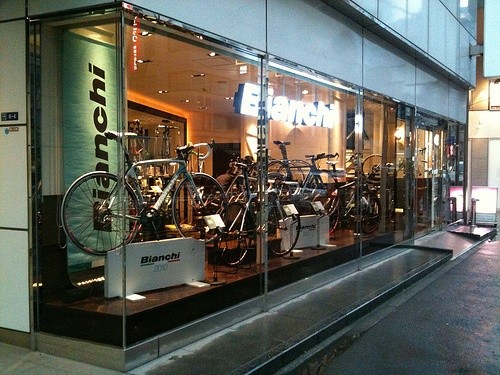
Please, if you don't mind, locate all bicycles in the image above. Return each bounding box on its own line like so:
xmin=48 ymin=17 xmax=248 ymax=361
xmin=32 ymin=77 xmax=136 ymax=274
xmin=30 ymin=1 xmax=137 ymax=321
xmin=60 ymin=129 xmax=456 ymax=275
